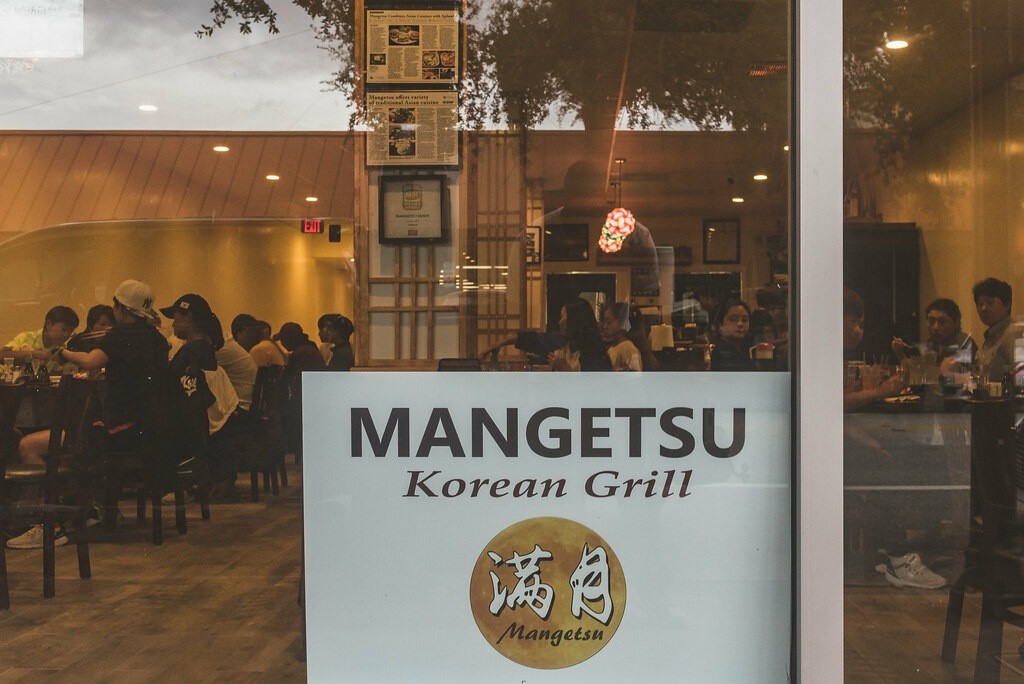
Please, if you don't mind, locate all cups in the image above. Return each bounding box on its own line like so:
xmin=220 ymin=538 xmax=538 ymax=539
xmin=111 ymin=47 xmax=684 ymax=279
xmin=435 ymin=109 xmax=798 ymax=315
xmin=969 ymin=375 xmax=987 ymax=400
xmin=481 ymin=355 xmax=495 ymax=372
xmin=954 ymin=350 xmax=971 ymax=390
xmin=902 ymin=355 xmax=937 ymax=384
xmin=987 ymin=382 xmax=1002 ymax=398
xmin=3 ymin=358 xmax=14 ymax=382
xmin=62 ymin=362 xmax=72 ymax=374
xmin=843 ymin=361 xmax=903 ymax=393
xmin=12 ymin=366 xmax=23 ymax=384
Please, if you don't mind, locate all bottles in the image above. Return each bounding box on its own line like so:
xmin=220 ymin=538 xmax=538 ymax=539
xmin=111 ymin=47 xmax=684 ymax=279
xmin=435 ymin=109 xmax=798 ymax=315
xmin=22 ymin=357 xmax=35 ymax=385
xmin=1002 ymin=373 xmax=1014 ymax=398
xmin=36 ymin=362 xmax=50 ymax=385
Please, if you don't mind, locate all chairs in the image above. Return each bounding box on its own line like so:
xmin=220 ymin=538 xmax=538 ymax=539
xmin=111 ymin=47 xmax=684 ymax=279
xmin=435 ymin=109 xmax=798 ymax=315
xmin=939 ymin=396 xmax=1024 ymax=684
xmin=0 ymin=363 xmax=290 ymax=611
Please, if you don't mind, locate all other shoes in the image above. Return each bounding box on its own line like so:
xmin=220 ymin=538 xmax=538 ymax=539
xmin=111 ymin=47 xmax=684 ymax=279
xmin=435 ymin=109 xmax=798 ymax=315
xmin=162 ymin=491 xmax=176 ymax=505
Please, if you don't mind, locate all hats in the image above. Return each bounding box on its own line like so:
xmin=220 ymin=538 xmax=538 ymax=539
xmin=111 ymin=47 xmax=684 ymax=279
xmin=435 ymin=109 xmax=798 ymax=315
xmin=114 ymin=280 xmax=160 ymax=322
xmin=274 ymin=322 xmax=303 ymax=341
xmin=158 ymin=294 xmax=211 ymax=322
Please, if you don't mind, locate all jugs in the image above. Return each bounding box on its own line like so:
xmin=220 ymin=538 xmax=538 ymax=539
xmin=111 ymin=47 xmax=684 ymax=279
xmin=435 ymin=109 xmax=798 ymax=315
xmin=750 ymin=343 xmax=776 ymax=360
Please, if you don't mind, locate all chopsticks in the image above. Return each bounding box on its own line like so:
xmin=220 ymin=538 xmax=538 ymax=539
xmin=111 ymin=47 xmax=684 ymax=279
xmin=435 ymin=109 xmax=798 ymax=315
xmin=83 ymin=331 xmax=106 ymax=341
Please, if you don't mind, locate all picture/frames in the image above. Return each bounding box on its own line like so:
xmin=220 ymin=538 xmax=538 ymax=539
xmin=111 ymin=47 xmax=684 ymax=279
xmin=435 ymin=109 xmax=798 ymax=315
xmin=377 ymin=174 xmax=449 ymax=244
xmin=526 ymin=226 xmax=542 ymax=264
xmin=702 ymin=218 xmax=740 ymax=265
xmin=545 ymin=223 xmax=589 ymax=261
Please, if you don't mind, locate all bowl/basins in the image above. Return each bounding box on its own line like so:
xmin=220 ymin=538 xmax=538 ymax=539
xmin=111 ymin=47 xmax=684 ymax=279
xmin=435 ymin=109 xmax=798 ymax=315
xmin=911 ymin=383 xmax=964 ymax=400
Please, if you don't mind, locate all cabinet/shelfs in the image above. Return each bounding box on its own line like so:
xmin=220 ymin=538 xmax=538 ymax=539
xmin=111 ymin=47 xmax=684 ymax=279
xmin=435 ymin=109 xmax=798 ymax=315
xmin=844 ymin=225 xmax=922 ymax=361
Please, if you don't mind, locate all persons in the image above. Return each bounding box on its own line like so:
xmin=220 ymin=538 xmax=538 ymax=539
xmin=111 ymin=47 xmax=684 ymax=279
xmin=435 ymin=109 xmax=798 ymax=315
xmin=510 ymin=158 xmax=662 ymax=372
xmin=0 ymin=279 xmax=354 ymax=550
xmin=478 ymin=287 xmax=1024 ymax=591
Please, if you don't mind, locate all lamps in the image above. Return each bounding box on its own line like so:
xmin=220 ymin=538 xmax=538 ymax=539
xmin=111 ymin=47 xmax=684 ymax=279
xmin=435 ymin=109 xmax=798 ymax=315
xmin=598 ymin=156 xmax=636 ymax=254
xmin=299 ymin=187 xmax=318 ymax=202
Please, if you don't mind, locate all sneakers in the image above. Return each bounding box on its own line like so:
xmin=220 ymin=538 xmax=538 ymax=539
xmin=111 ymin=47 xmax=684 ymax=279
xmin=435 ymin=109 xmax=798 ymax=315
xmin=6 ymin=523 xmax=68 ymax=549
xmin=65 ymin=505 xmax=101 ymax=533
xmin=871 ymin=546 xmax=888 ymax=573
xmin=884 ymin=553 xmax=947 ymax=590
